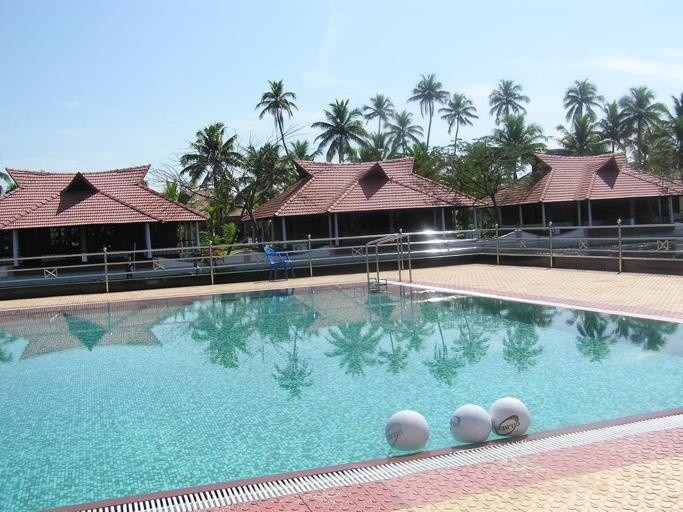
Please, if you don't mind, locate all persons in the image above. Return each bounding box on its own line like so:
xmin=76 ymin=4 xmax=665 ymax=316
xmin=126 ymin=256 xmax=135 ymax=280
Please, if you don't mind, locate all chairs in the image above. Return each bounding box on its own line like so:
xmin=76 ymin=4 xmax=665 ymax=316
xmin=264 ymin=247 xmax=295 ymax=281
